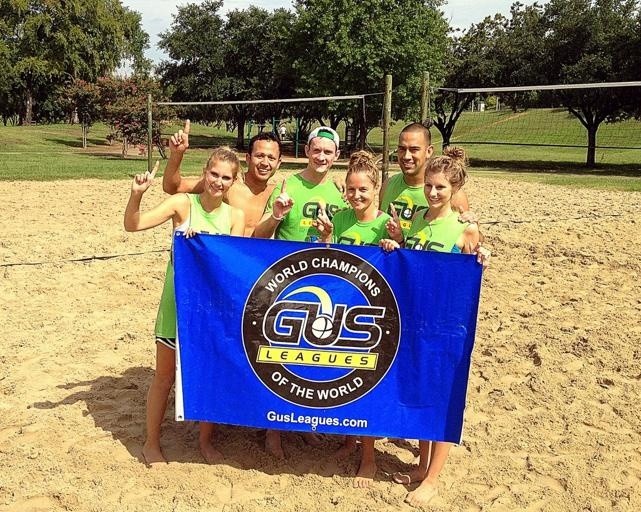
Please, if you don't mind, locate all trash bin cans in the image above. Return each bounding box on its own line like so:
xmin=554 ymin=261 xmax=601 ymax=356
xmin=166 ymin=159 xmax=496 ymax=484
xmin=70 ymin=107 xmax=80 ymax=124
xmin=477 ymin=100 xmax=485 ymax=112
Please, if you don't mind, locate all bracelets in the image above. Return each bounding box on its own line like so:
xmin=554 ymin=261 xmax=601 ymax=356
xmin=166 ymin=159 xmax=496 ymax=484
xmin=398 ymin=239 xmax=405 ymax=244
xmin=272 ymin=213 xmax=286 ymax=221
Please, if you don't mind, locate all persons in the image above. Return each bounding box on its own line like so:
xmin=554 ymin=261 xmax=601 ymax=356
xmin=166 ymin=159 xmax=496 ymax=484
xmin=252 ymin=126 xmax=354 ymax=459
xmin=124 ymin=144 xmax=248 ymax=473
xmin=383 ymin=145 xmax=493 ymax=508
xmin=279 ymin=125 xmax=288 ymax=141
xmin=378 ymin=122 xmax=476 ymax=226
xmin=310 ymin=151 xmax=403 ymax=489
xmin=161 ymin=119 xmax=352 ymax=240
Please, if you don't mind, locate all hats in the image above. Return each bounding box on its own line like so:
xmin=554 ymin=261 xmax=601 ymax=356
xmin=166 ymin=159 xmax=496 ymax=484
xmin=307 ymin=126 xmax=340 ymax=151
